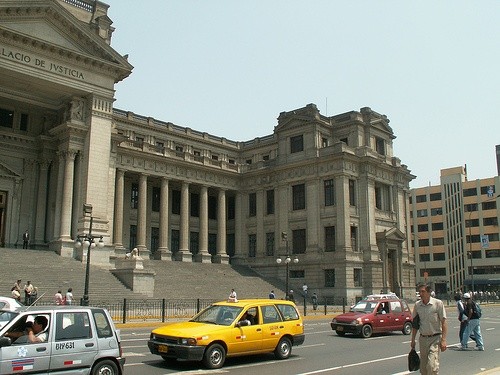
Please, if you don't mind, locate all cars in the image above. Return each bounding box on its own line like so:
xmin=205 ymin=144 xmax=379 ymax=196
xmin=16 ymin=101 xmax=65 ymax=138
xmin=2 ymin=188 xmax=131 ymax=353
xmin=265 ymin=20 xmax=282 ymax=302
xmin=0 ymin=306 xmax=126 ymax=375
xmin=148 ymin=298 xmax=306 ymax=369
xmin=331 ymin=298 xmax=413 ymax=338
xmin=0 ymin=296 xmax=73 ymax=337
xmin=348 ymin=294 xmax=400 ymax=311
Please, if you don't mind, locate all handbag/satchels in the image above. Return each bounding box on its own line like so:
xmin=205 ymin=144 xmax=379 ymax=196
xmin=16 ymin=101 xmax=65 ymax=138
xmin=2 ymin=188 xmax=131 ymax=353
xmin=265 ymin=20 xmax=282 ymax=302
xmin=412 ymin=315 xmax=419 ymax=330
xmin=408 ymin=350 xmax=420 ymax=371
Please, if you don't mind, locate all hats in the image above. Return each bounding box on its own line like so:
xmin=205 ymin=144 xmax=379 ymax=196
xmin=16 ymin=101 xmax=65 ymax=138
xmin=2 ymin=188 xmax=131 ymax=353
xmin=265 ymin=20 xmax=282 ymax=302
xmin=464 ymin=293 xmax=470 ymax=298
xmin=290 ymin=290 xmax=293 ymax=293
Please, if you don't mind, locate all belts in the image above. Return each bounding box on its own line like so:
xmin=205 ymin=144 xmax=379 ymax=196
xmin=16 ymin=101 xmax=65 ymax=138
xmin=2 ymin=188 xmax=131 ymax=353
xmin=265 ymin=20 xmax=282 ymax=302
xmin=420 ymin=333 xmax=440 ymax=337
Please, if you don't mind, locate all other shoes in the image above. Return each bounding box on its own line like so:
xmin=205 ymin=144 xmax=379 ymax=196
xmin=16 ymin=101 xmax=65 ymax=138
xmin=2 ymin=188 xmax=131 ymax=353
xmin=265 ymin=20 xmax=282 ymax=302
xmin=474 ymin=347 xmax=484 ymax=351
xmin=457 ymin=347 xmax=467 ymax=350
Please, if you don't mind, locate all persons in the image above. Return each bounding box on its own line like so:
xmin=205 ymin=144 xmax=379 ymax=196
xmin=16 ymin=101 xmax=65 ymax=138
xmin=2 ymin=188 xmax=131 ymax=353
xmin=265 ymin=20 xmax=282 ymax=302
xmin=378 ymin=305 xmax=385 ymax=313
xmin=3 ymin=315 xmax=49 ymax=343
xmin=54 ymin=288 xmax=75 ymax=306
xmin=410 ymin=283 xmax=448 ymax=375
xmin=288 ymin=289 xmax=295 ymax=304
xmin=11 ymin=280 xmax=38 ymax=306
xmin=381 ymin=290 xmax=384 ymax=294
xmin=269 ymin=291 xmax=275 ymax=299
xmin=302 ymin=283 xmax=318 ymax=310
xmin=224 ymin=311 xmax=251 ymax=326
xmin=229 ymin=289 xmax=236 ymax=298
xmin=430 ymin=290 xmax=500 ymax=352
xmin=23 ymin=230 xmax=30 ymax=249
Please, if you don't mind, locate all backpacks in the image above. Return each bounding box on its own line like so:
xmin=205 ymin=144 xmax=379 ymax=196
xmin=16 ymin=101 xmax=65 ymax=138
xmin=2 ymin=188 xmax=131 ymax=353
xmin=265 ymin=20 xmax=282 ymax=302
xmin=470 ymin=301 xmax=481 ymax=318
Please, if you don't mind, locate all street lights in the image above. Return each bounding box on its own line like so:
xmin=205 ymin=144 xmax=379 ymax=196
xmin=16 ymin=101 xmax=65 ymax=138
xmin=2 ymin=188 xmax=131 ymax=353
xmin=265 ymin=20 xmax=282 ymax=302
xmin=276 ymin=231 xmax=300 ymax=300
xmin=76 ymin=203 xmax=103 ymax=306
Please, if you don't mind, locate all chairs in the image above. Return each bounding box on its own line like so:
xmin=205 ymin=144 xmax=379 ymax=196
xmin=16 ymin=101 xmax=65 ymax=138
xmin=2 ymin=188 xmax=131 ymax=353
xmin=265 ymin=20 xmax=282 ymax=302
xmin=224 ymin=313 xmax=233 ymax=319
xmin=246 ymin=314 xmax=255 ymax=325
